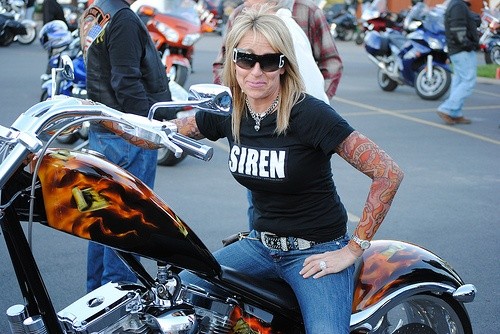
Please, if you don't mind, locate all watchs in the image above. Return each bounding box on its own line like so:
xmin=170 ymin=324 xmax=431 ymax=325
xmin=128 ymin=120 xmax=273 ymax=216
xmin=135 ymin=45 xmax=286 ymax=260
xmin=351 ymin=235 xmax=371 ymax=250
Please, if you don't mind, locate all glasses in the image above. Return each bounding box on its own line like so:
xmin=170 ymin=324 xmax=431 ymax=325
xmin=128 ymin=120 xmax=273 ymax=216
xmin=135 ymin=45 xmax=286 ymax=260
xmin=232 ymin=47 xmax=285 ymax=72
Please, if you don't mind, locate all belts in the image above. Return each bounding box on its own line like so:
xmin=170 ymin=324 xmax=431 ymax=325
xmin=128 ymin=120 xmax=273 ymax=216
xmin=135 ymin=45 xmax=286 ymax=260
xmin=257 ymin=231 xmax=345 ymax=251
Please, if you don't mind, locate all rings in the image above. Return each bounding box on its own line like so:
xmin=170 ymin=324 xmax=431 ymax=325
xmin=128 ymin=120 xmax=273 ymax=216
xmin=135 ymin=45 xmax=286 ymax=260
xmin=319 ymin=261 xmax=326 ymax=270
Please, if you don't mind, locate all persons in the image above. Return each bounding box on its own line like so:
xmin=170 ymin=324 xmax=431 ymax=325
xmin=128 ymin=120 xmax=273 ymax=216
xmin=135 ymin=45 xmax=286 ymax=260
xmin=45 ymin=15 xmax=403 ymax=334
xmin=77 ymin=0 xmax=178 ymax=294
xmin=42 ymin=0 xmax=70 ymax=43
xmin=212 ymin=0 xmax=344 ymax=232
xmin=436 ymin=0 xmax=480 ymax=124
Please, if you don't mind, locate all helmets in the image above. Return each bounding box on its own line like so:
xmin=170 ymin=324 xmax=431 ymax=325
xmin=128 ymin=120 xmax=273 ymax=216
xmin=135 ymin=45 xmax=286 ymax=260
xmin=38 ymin=19 xmax=70 ymax=51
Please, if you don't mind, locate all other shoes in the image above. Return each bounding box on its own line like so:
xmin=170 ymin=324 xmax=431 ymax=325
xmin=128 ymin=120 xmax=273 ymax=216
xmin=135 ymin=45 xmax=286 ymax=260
xmin=453 ymin=116 xmax=473 ymax=125
xmin=438 ymin=110 xmax=457 ymax=123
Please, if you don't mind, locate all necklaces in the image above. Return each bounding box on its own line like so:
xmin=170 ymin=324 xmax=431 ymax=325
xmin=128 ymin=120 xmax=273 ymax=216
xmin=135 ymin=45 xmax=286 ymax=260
xmin=87 ymin=99 xmax=95 ymax=106
xmin=245 ymin=93 xmax=279 ymax=132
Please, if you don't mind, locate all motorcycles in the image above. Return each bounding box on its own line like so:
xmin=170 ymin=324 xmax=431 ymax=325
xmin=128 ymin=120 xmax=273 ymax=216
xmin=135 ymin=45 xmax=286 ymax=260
xmin=135 ymin=1 xmax=215 ymax=168
xmin=477 ymin=0 xmax=500 ymax=67
xmin=0 ymin=53 xmax=479 ymax=334
xmin=0 ymin=0 xmax=39 ymax=48
xmin=324 ymin=0 xmax=454 ymax=103
